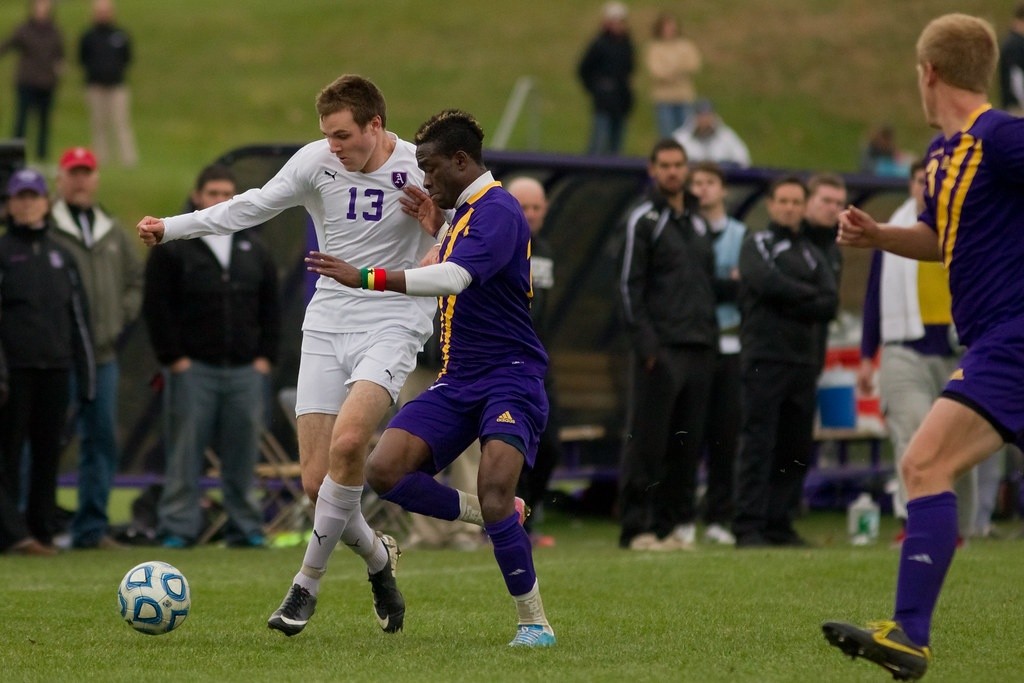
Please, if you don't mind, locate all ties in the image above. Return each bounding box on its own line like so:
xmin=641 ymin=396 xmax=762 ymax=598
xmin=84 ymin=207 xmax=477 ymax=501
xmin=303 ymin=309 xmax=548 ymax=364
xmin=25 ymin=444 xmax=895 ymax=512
xmin=79 ymin=211 xmax=92 ymax=248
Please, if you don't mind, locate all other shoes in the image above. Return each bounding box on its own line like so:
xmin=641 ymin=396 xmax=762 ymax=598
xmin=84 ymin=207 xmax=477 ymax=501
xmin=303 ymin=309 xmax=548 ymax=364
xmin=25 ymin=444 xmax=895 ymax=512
xmin=10 ymin=538 xmax=57 ymax=555
xmin=631 ymin=533 xmax=694 ymax=552
xmin=703 ymin=524 xmax=735 ymax=545
xmin=72 ymin=536 xmax=124 ymax=550
xmin=888 ymin=529 xmax=964 ymax=549
xmin=226 ymin=531 xmax=262 ymax=546
xmin=161 ymin=534 xmax=188 ymax=549
xmin=674 ymin=522 xmax=696 ymax=545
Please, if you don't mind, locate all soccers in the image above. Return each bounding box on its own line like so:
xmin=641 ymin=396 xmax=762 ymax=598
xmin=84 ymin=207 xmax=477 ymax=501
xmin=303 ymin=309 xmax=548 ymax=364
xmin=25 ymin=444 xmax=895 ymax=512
xmin=117 ymin=561 xmax=193 ymax=636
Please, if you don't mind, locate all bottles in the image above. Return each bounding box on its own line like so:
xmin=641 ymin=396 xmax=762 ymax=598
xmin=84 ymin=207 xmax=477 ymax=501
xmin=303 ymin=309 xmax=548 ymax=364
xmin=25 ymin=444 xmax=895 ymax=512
xmin=848 ymin=492 xmax=880 ymax=545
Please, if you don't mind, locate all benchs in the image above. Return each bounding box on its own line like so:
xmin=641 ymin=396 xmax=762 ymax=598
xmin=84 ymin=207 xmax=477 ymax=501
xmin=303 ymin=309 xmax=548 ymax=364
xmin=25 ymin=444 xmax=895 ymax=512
xmin=549 ymin=349 xmax=891 ymax=442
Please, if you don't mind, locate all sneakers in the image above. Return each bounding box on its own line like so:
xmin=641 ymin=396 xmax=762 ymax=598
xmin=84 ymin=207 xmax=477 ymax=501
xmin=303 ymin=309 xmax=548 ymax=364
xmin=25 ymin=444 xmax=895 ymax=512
xmin=508 ymin=625 xmax=556 ymax=648
xmin=268 ymin=583 xmax=318 ymax=636
xmin=514 ymin=497 xmax=531 ymax=528
xmin=368 ymin=531 xmax=406 ymax=633
xmin=822 ymin=619 xmax=932 ymax=681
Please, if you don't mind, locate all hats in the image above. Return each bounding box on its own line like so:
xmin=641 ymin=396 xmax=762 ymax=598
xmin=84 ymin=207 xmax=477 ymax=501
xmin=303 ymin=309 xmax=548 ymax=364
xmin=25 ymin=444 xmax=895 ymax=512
xmin=61 ymin=148 xmax=96 ymax=172
xmin=8 ymin=168 xmax=45 ymax=196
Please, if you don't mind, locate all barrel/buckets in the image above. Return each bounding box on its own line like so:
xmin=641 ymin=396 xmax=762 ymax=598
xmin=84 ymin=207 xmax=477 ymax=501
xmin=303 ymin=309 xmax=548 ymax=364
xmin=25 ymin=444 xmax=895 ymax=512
xmin=819 ymin=371 xmax=856 ymax=428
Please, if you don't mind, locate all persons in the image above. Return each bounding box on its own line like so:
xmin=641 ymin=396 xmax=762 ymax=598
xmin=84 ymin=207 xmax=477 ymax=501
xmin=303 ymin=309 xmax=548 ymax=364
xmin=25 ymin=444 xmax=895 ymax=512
xmin=821 ymin=3 xmax=1024 ymax=683
xmin=303 ymin=107 xmax=562 ymax=651
xmin=0 ymin=0 xmax=64 ymax=161
xmin=645 ymin=11 xmax=751 ymax=169
xmin=0 ymin=142 xmax=100 ymax=560
xmin=735 ymin=171 xmax=846 ymax=548
xmin=618 ymin=136 xmax=751 ymax=554
xmin=45 ymin=148 xmax=132 ymax=552
xmin=135 ymin=70 xmax=473 ymax=637
xmin=574 ymin=0 xmax=638 ymax=156
xmin=143 ymin=165 xmax=284 ymax=551
xmin=75 ymin=0 xmax=140 ymax=168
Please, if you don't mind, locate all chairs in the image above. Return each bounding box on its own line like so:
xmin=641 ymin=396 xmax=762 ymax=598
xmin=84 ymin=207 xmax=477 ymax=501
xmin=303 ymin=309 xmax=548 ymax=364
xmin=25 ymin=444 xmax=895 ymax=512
xmin=52 ymin=392 xmax=453 ymax=546
